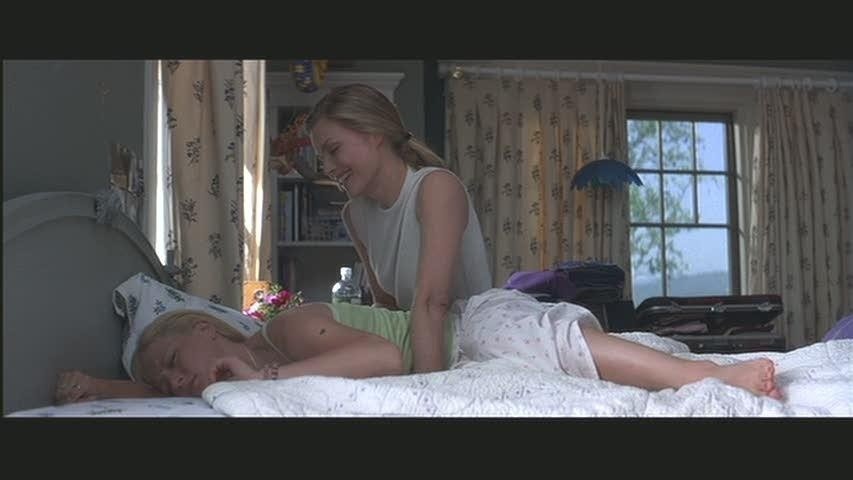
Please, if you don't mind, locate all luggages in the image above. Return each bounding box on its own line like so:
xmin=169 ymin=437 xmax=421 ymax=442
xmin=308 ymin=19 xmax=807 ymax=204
xmin=635 ymin=294 xmax=786 ymax=354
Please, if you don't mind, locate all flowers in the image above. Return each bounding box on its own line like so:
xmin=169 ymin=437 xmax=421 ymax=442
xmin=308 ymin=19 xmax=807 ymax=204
xmin=243 ymin=282 xmax=306 ymax=323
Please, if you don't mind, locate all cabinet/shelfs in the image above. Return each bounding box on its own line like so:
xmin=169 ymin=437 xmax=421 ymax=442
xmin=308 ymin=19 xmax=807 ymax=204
xmin=264 ymin=72 xmax=406 ymax=302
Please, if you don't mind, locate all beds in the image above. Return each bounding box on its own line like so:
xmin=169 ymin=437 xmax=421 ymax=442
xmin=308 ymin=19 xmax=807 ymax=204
xmin=2 ymin=191 xmax=853 ymax=417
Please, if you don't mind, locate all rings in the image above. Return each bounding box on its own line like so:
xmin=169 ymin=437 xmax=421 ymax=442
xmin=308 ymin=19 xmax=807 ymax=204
xmin=70 ymin=383 xmax=81 ymax=391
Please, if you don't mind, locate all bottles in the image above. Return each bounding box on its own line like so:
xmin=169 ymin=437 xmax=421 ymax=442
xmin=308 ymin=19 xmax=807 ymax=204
xmin=331 ymin=267 xmax=364 ymax=307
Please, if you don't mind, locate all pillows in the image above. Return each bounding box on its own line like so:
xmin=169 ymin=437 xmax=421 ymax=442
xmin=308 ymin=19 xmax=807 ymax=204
xmin=111 ymin=272 xmax=265 ymax=383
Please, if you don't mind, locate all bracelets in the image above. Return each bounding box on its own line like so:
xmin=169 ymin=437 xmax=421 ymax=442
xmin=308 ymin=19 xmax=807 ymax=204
xmin=271 ymin=362 xmax=280 ymax=380
xmin=263 ymin=363 xmax=269 ymax=378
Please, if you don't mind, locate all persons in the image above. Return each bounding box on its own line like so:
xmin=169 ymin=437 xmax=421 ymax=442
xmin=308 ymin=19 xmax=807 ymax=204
xmin=54 ymin=282 xmax=786 ymax=405
xmin=305 ymin=82 xmax=495 ymax=374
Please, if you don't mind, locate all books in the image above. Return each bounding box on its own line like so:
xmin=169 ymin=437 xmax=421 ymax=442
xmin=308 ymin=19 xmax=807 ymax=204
xmin=279 ymin=259 xmax=304 ymax=291
xmin=279 ymin=181 xmax=313 ymax=242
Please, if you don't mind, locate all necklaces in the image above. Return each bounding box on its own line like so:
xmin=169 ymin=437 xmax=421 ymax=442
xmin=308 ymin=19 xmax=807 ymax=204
xmin=240 ymin=341 xmax=258 ymax=366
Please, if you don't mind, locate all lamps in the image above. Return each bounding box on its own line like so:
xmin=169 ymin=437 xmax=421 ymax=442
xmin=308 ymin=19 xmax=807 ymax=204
xmin=570 ymin=152 xmax=645 ymax=264
